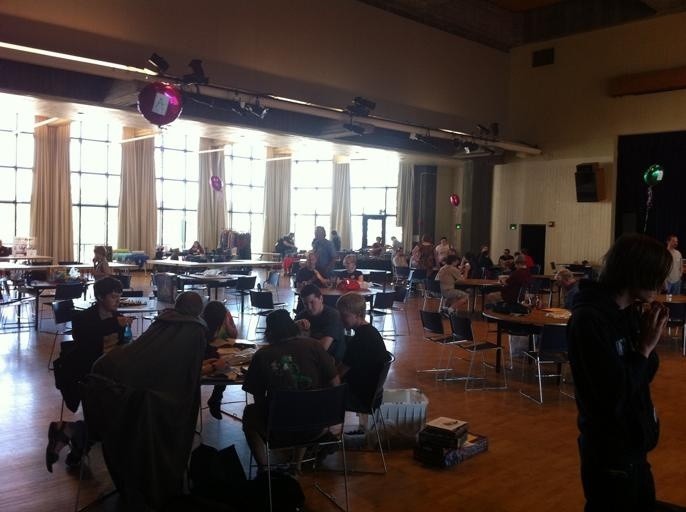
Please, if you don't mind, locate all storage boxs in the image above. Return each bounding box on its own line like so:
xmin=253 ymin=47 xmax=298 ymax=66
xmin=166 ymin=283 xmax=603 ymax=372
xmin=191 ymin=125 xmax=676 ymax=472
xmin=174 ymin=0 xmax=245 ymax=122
xmin=377 ymin=388 xmax=428 ymax=447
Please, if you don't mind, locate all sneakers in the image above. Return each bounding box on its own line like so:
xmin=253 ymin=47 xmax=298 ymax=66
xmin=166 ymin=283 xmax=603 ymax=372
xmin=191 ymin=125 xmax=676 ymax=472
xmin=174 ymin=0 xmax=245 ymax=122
xmin=346 ymin=428 xmax=369 ymax=438
xmin=441 ymin=306 xmax=454 ymax=319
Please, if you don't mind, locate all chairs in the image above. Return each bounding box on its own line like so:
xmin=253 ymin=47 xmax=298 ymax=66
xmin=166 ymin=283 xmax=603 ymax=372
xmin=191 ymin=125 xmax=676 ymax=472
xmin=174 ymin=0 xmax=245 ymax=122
xmin=141 ymin=272 xmax=178 ymax=334
xmin=250 ymin=290 xmax=287 ymax=333
xmin=392 ymin=265 xmax=411 ymax=287
xmin=233 ymin=277 xmax=256 ymax=313
xmin=381 ymin=288 xmax=411 ymax=336
xmin=22 ymin=271 xmax=47 ymax=298
xmin=403 ymin=267 xmax=432 ymax=302
xmin=262 ymin=271 xmax=280 ymax=302
xmin=366 ymin=292 xmax=397 ymax=342
xmin=112 ymin=274 xmax=134 ymax=292
xmin=518 ymin=322 xmax=570 ymax=407
xmin=445 ymin=314 xmax=507 ymax=390
xmin=417 ymin=310 xmax=486 ymax=381
xmin=424 ymin=280 xmax=447 ymax=313
xmin=121 ymin=290 xmax=142 ymax=332
xmin=40 ymin=285 xmax=85 ymax=335
xmin=311 ymin=353 xmax=395 ymax=474
xmin=48 ymin=299 xmax=75 ymax=370
xmin=370 ymin=271 xmax=387 ymax=293
xmin=248 ymin=384 xmax=350 ymax=512
xmin=492 ymin=281 xmax=534 ymax=314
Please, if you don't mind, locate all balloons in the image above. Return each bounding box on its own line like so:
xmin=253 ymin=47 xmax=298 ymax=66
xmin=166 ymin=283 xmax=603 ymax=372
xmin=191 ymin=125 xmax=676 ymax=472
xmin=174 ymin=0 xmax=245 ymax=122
xmin=643 ymin=165 xmax=664 ymax=187
xmin=211 ymin=176 xmax=223 ymax=191
xmin=138 ymin=79 xmax=183 ymax=127
xmin=450 ymin=193 xmax=461 ymax=208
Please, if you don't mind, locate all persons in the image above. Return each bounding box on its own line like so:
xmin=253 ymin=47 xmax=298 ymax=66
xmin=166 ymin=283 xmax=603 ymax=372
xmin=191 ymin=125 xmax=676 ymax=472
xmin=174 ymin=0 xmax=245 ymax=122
xmin=297 ymin=251 xmax=329 ymax=288
xmin=203 ymin=300 xmax=237 ymax=420
xmin=294 ymin=284 xmax=341 ymax=350
xmin=664 ymin=235 xmax=681 ymax=324
xmin=337 ymin=254 xmax=363 ymax=287
xmin=521 ymin=248 xmax=534 ymax=267
xmin=332 ymin=230 xmax=341 ymax=251
xmin=92 ymin=246 xmax=111 ymax=280
xmin=435 ymin=255 xmax=471 ymax=317
xmin=45 ymin=291 xmax=210 ymax=473
xmin=282 ymin=233 xmax=296 ymax=256
xmin=331 ymin=292 xmax=388 ymax=405
xmin=497 ymin=248 xmax=514 ymax=266
xmin=555 ymin=269 xmax=579 ymax=311
xmin=441 ymin=248 xmax=460 ymax=266
xmin=72 ymin=277 xmax=134 ymax=397
xmin=151 ymin=246 xmax=164 ymax=274
xmin=391 ymin=236 xmax=400 ymax=248
xmin=412 ymin=233 xmax=439 ymax=273
xmin=569 ymin=231 xmax=669 ymax=510
xmin=241 ymin=310 xmax=339 ymax=484
xmin=488 ymin=254 xmax=530 ymax=310
xmin=190 ymin=241 xmax=205 ymax=256
xmin=313 ymin=226 xmax=337 ymax=283
xmin=374 ymin=236 xmax=382 ymax=249
xmin=479 ymin=245 xmax=488 ymax=252
xmin=394 ymin=247 xmax=409 ymax=266
xmin=436 ymin=236 xmax=454 ymax=262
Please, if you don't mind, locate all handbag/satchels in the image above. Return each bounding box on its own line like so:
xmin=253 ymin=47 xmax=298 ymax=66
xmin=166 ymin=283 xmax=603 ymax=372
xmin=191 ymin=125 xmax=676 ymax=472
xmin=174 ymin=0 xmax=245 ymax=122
xmin=250 ymin=284 xmax=274 ymax=309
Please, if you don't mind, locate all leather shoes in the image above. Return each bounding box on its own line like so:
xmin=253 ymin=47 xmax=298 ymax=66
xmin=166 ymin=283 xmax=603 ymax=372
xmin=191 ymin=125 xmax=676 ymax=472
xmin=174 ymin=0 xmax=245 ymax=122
xmin=208 ymin=397 xmax=224 ymax=420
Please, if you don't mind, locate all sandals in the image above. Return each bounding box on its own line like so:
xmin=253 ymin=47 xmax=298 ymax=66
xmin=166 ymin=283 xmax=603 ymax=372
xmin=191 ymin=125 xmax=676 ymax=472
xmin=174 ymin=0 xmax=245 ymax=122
xmin=66 ymin=450 xmax=86 ymax=470
xmin=46 ymin=420 xmax=65 ymax=473
